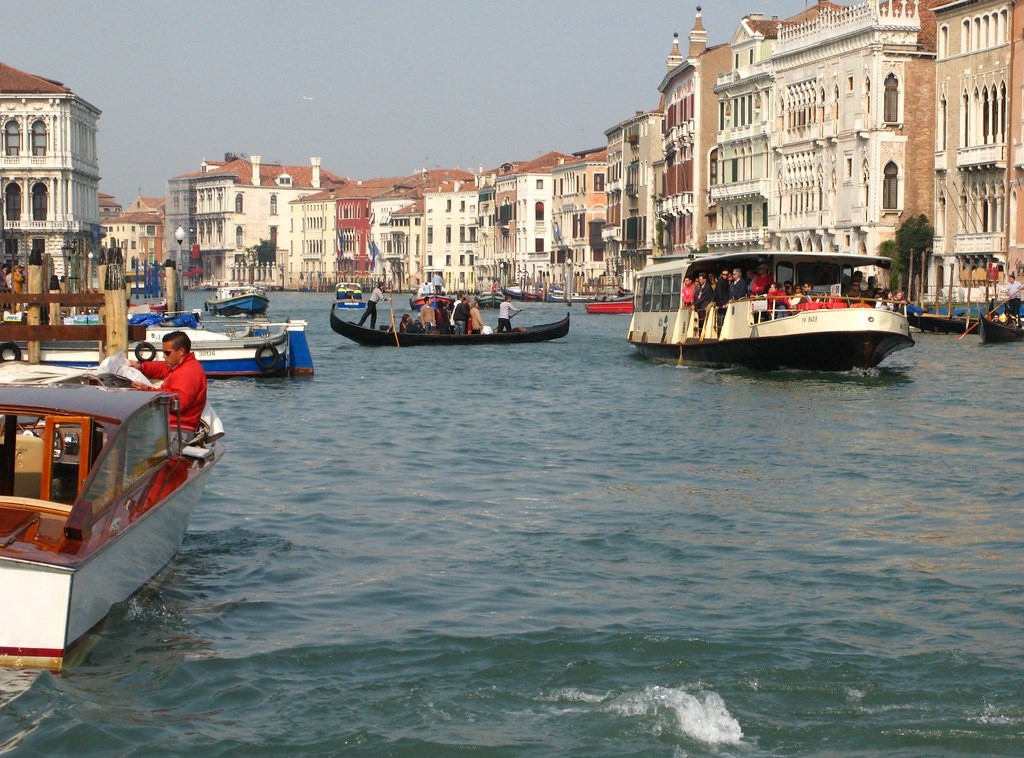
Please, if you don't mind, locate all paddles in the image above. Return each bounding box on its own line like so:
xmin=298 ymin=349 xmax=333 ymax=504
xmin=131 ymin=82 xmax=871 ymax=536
xmin=386 ymin=291 xmax=401 ymax=348
xmin=957 ymin=284 xmax=1024 ymax=340
xmin=493 ymin=308 xmax=522 ymax=331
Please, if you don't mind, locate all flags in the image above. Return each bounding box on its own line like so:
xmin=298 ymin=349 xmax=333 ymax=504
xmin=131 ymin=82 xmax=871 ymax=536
xmin=337 ymin=230 xmax=344 ymax=257
xmin=367 ymin=241 xmax=379 ymax=268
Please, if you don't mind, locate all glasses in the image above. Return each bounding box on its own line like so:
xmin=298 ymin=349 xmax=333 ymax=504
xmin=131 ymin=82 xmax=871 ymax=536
xmin=163 ymin=346 xmax=182 ymax=356
xmin=852 ymin=285 xmax=860 ymax=286
xmin=723 ymin=274 xmax=729 ymax=276
xmin=733 ymin=274 xmax=737 ymax=276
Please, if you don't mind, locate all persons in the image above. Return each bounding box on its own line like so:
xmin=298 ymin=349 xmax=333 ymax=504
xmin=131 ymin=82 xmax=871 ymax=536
xmin=0 ymin=262 xmax=25 ymax=312
xmin=497 ymin=295 xmax=520 ymax=332
xmin=358 ymin=281 xmax=392 ymax=329
xmin=423 ymin=282 xmax=431 ymax=296
xmin=433 ymin=272 xmax=443 ymax=294
xmin=491 ymin=280 xmax=503 ymax=296
xmin=399 ymin=295 xmax=484 ymax=335
xmin=1004 ymin=275 xmax=1024 ymax=322
xmin=129 ymin=332 xmax=207 ymax=513
xmin=846 ymin=271 xmax=910 ymax=313
xmin=680 ymin=264 xmax=815 ymax=320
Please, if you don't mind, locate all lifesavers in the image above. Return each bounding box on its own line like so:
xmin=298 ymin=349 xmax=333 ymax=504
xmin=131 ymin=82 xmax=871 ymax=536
xmin=0 ymin=343 xmax=22 ymax=364
xmin=204 ymin=302 xmax=208 ymax=311
xmin=134 ymin=342 xmax=156 ymax=362
xmin=255 ymin=342 xmax=280 ymax=370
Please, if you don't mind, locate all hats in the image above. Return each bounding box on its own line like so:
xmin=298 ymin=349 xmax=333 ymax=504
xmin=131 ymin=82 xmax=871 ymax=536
xmin=757 ymin=264 xmax=769 ymax=269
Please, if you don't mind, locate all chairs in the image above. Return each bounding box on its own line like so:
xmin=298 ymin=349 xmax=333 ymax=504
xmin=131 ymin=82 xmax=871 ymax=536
xmin=0 ymin=434 xmax=44 ymax=498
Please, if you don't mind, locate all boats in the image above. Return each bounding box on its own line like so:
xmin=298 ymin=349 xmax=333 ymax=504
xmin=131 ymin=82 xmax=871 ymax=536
xmin=0 ymin=357 xmax=228 ymax=675
xmin=475 ymin=283 xmax=634 ymax=307
xmin=625 ymin=249 xmax=917 ymax=376
xmin=204 ymin=280 xmax=271 ymax=317
xmin=335 ymin=282 xmax=367 ymax=308
xmin=329 ymin=302 xmax=570 ymax=348
xmin=907 ymin=296 xmax=1024 ymax=344
xmin=583 ymin=301 xmax=634 ymax=314
xmin=0 ymin=313 xmax=316 ymax=380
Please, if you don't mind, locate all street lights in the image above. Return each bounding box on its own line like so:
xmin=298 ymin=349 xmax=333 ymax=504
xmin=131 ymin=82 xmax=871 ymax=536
xmin=174 ymin=224 xmax=186 ymax=301
xmin=566 ymin=258 xmax=573 ymax=307
xmin=499 ymin=262 xmax=504 ymax=288
